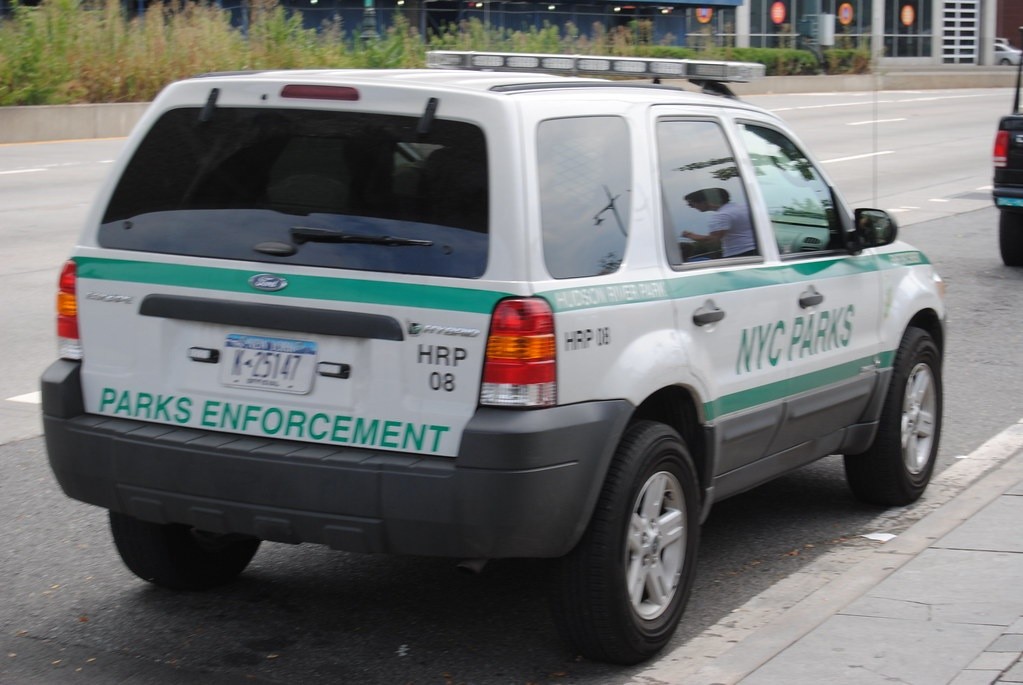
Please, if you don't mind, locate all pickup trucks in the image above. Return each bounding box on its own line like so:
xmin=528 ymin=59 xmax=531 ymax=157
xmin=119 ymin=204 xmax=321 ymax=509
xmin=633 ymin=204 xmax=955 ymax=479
xmin=991 ymin=26 xmax=1023 ymax=269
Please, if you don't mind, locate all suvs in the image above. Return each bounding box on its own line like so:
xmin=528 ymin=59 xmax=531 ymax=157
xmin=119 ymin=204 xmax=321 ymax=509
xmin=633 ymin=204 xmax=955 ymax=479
xmin=34 ymin=46 xmax=950 ymax=669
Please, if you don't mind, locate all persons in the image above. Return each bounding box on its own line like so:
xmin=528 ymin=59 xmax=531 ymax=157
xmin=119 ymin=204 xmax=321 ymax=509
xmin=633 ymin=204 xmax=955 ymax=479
xmin=681 ymin=187 xmax=759 ymax=258
